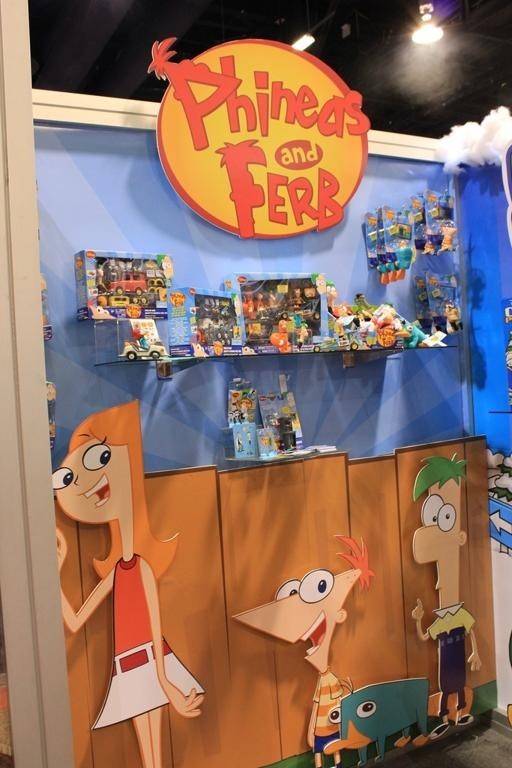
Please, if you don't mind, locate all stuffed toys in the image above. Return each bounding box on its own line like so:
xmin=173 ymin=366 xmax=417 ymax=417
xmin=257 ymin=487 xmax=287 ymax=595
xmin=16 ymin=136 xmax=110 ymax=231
xmin=73 ymin=189 xmax=458 ymax=365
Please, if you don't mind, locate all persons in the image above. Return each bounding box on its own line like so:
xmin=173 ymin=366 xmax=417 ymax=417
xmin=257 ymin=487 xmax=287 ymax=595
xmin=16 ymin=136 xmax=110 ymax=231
xmin=133 ymin=328 xmax=153 ymax=349
xmin=242 ymin=287 xmax=316 ymax=320
